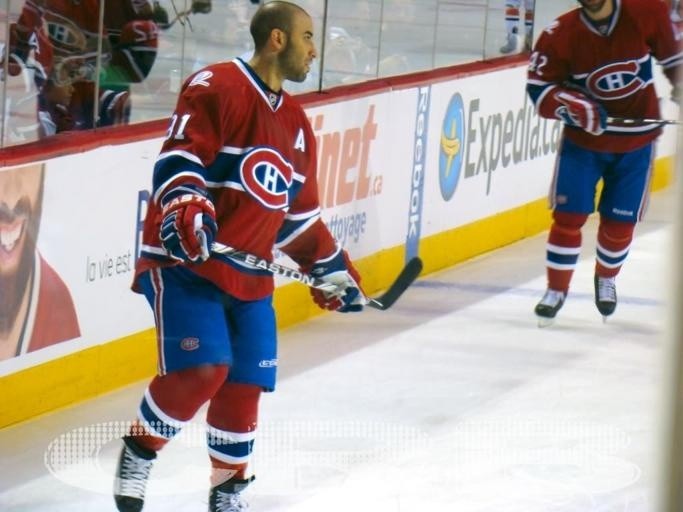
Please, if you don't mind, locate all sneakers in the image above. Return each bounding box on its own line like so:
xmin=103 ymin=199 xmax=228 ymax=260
xmin=595 ymin=276 xmax=618 ymax=316
xmin=501 ymin=41 xmax=518 ymax=53
xmin=536 ymin=286 xmax=568 ymax=316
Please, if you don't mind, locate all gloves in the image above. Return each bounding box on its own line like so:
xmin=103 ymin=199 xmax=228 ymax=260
xmin=160 ymin=185 xmax=216 ymax=265
xmin=302 ymin=241 xmax=364 ymax=312
xmin=553 ymin=92 xmax=606 ymax=136
xmin=5 ymin=22 xmax=31 ymax=76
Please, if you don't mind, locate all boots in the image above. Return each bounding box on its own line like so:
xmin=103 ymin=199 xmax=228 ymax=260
xmin=209 ymin=473 xmax=255 ymax=511
xmin=113 ymin=433 xmax=155 ymax=511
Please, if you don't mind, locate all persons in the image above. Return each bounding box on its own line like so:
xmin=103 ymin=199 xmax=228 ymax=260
xmin=34 ymin=62 xmax=79 ymax=136
xmin=5 ymin=0 xmax=160 ymax=138
xmin=110 ymin=2 xmax=370 ymax=510
xmin=0 ymin=161 xmax=81 ymax=360
xmin=526 ymin=1 xmax=681 ymax=322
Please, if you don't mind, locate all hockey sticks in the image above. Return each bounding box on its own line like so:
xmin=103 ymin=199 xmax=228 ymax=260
xmin=211 ymin=240 xmax=423 ymax=310
